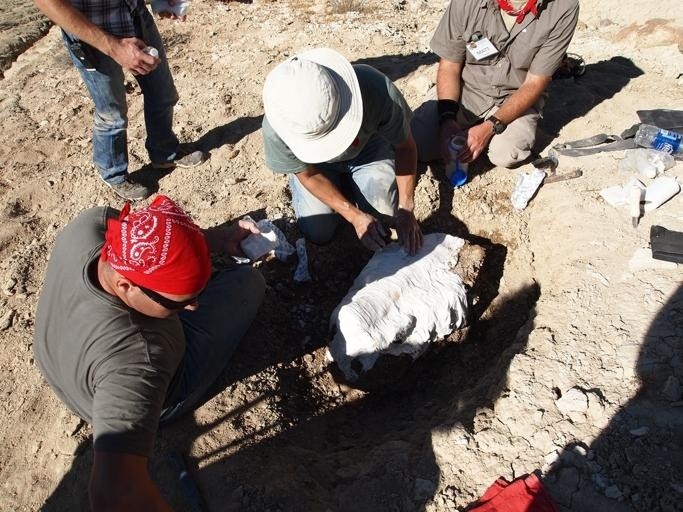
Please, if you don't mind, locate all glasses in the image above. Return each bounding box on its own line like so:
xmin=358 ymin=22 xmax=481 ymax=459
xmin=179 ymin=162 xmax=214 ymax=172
xmin=139 ymin=286 xmax=206 ymax=310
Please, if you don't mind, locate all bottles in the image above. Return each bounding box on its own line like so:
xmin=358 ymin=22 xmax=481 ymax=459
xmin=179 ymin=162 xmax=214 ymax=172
xmin=633 ymin=124 xmax=682 ymax=157
xmin=142 ymin=46 xmax=160 ymax=60
xmin=444 ymin=134 xmax=470 ymax=185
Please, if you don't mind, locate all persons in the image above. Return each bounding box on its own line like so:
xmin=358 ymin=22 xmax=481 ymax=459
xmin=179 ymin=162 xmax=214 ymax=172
xmin=33 ymin=0 xmax=205 ymax=201
xmin=33 ymin=194 xmax=270 ymax=511
xmin=410 ymin=0 xmax=580 ymax=169
xmin=262 ymin=48 xmax=425 ymax=280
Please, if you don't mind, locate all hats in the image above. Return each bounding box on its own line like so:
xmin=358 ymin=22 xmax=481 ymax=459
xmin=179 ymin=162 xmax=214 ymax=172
xmin=262 ymin=48 xmax=364 ymax=164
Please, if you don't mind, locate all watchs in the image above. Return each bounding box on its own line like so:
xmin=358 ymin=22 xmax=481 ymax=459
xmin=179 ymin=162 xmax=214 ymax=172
xmin=486 ymin=115 xmax=505 ymax=135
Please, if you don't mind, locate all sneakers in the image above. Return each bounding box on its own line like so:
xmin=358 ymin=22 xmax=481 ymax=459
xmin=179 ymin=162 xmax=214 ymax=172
xmin=99 ymin=174 xmax=149 ymax=201
xmin=151 ymin=149 xmax=206 ymax=169
